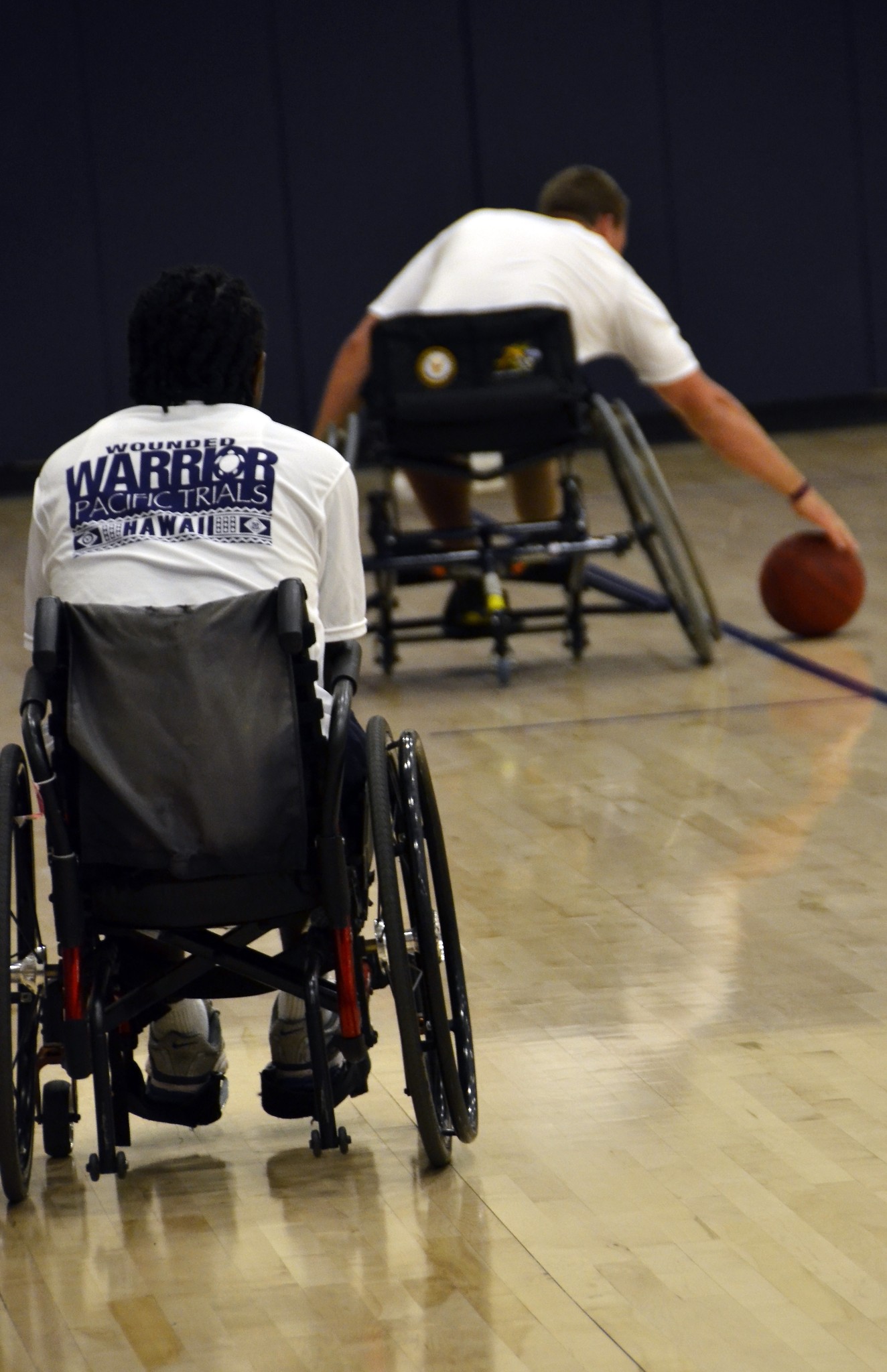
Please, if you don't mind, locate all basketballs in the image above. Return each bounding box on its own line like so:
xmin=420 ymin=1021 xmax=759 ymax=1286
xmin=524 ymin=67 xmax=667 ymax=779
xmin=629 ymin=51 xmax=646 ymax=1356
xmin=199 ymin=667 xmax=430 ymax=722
xmin=759 ymin=531 xmax=866 ymax=636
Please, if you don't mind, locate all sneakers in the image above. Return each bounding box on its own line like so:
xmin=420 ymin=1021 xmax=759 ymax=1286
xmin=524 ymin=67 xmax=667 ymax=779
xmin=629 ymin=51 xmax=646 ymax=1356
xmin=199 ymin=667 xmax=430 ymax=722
xmin=146 ymin=999 xmax=228 ymax=1094
xmin=269 ymin=995 xmax=343 ymax=1077
xmin=441 ymin=573 xmax=513 ymax=633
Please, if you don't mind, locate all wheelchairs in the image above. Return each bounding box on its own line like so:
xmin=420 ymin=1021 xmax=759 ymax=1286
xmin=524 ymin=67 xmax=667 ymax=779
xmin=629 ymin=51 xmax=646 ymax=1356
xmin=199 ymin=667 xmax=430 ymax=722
xmin=324 ymin=304 xmax=722 ymax=675
xmin=0 ymin=578 xmax=479 ymax=1205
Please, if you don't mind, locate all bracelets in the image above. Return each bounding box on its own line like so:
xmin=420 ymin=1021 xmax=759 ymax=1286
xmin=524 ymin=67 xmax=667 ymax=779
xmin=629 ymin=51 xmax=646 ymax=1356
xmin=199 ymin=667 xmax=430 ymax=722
xmin=791 ymin=477 xmax=816 ymax=505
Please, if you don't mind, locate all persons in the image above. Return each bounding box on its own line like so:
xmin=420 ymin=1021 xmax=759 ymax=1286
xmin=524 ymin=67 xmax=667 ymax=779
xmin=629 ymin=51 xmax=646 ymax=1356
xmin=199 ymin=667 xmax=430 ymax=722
xmin=310 ymin=162 xmax=862 ymax=643
xmin=20 ymin=262 xmax=372 ymax=1094
